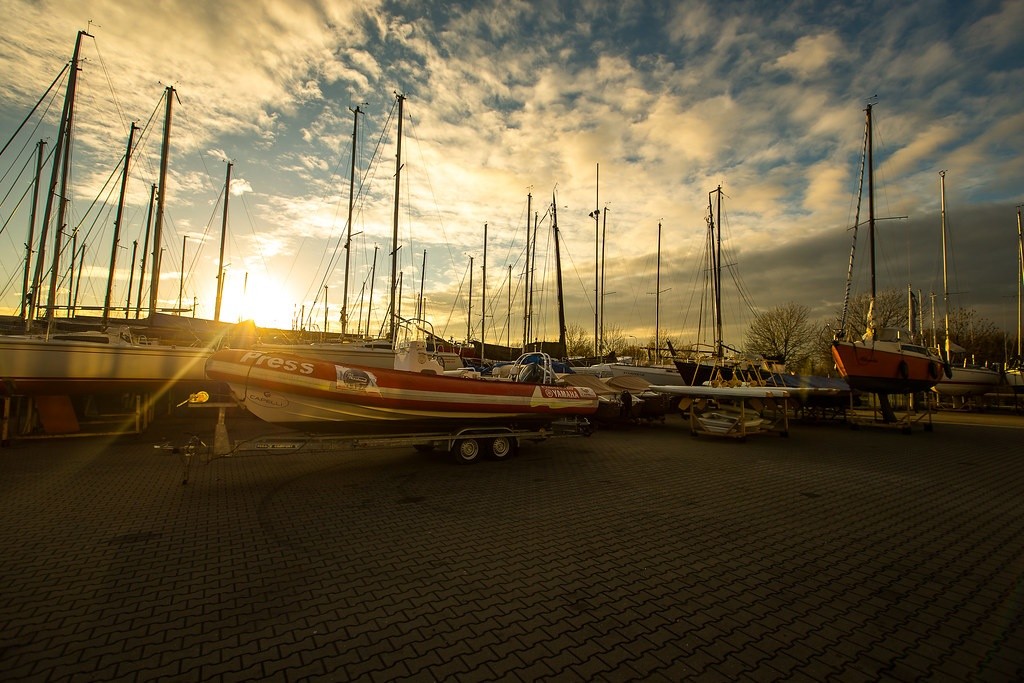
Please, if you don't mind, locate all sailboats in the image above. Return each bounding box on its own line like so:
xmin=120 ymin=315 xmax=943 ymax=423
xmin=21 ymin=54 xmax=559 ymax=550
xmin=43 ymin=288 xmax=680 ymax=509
xmin=0 ymin=19 xmax=1024 ymax=432
